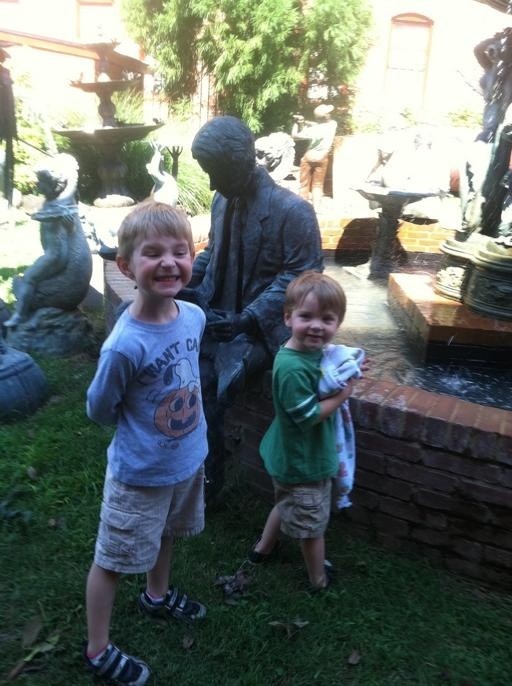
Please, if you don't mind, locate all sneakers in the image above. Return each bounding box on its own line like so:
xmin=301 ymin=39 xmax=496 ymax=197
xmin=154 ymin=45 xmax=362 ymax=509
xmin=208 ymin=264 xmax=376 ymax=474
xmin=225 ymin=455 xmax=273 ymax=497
xmin=82 ymin=640 xmax=156 ymax=686
xmin=247 ymin=538 xmax=284 ymax=566
xmin=138 ymin=582 xmax=206 ymax=624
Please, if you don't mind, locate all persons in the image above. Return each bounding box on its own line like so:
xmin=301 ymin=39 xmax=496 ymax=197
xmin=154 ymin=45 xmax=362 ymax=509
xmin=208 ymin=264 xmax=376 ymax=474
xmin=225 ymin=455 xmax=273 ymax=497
xmin=83 ymin=201 xmax=210 ymax=686
xmin=292 ymin=103 xmax=338 ymax=223
xmin=177 ymin=116 xmax=324 ymax=516
xmin=249 ymin=273 xmax=358 ymax=588
xmin=2 ymin=170 xmax=68 ymax=330
